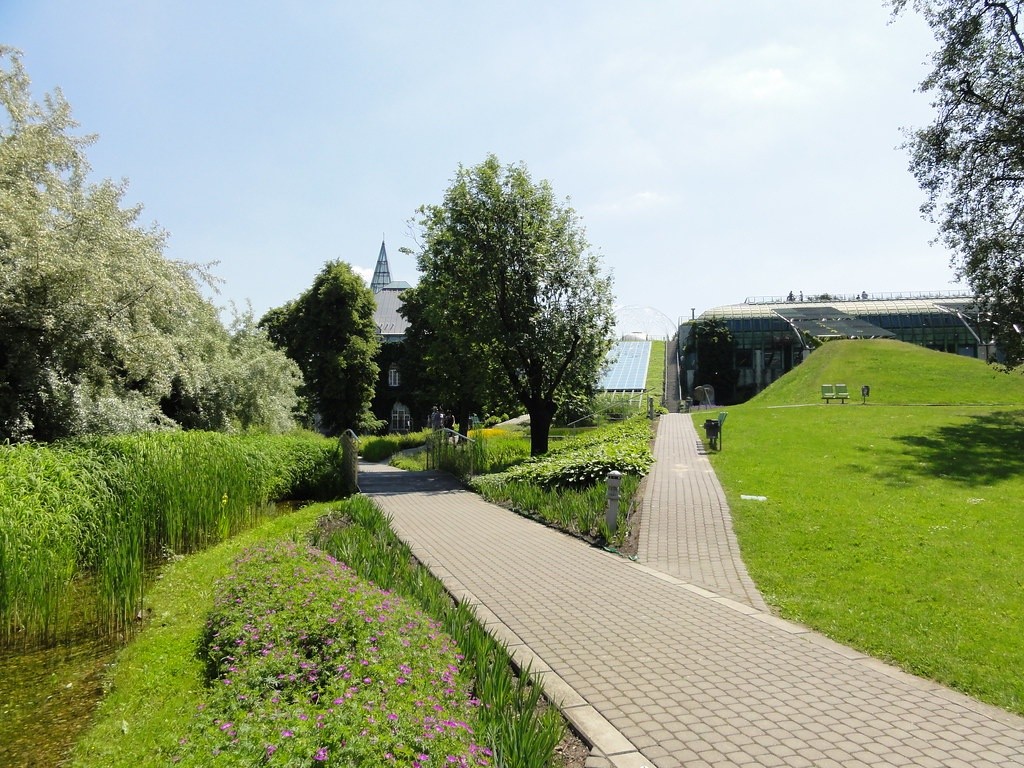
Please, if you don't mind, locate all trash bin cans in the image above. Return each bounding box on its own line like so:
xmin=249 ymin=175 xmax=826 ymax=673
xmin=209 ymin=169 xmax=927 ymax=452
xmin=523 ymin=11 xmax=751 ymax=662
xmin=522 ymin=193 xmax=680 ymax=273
xmin=861 ymin=385 xmax=870 ymax=397
xmin=705 ymin=420 xmax=719 ymax=440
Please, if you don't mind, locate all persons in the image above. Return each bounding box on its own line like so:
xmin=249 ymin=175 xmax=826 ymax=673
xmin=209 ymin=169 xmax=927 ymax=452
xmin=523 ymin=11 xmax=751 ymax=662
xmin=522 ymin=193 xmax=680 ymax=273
xmin=427 ymin=405 xmax=456 ymax=435
xmin=789 ymin=291 xmax=868 ymax=302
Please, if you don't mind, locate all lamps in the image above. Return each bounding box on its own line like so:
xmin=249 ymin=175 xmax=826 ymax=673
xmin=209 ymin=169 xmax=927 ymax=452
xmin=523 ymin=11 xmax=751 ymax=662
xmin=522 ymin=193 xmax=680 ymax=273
xmin=606 ymin=470 xmax=622 ymax=500
xmin=649 ymin=397 xmax=654 ymax=408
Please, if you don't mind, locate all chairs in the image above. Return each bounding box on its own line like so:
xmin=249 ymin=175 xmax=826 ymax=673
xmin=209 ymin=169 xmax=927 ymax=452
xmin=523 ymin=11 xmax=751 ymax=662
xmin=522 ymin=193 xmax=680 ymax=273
xmin=834 ymin=384 xmax=850 ymax=404
xmin=821 ymin=385 xmax=835 ymax=403
